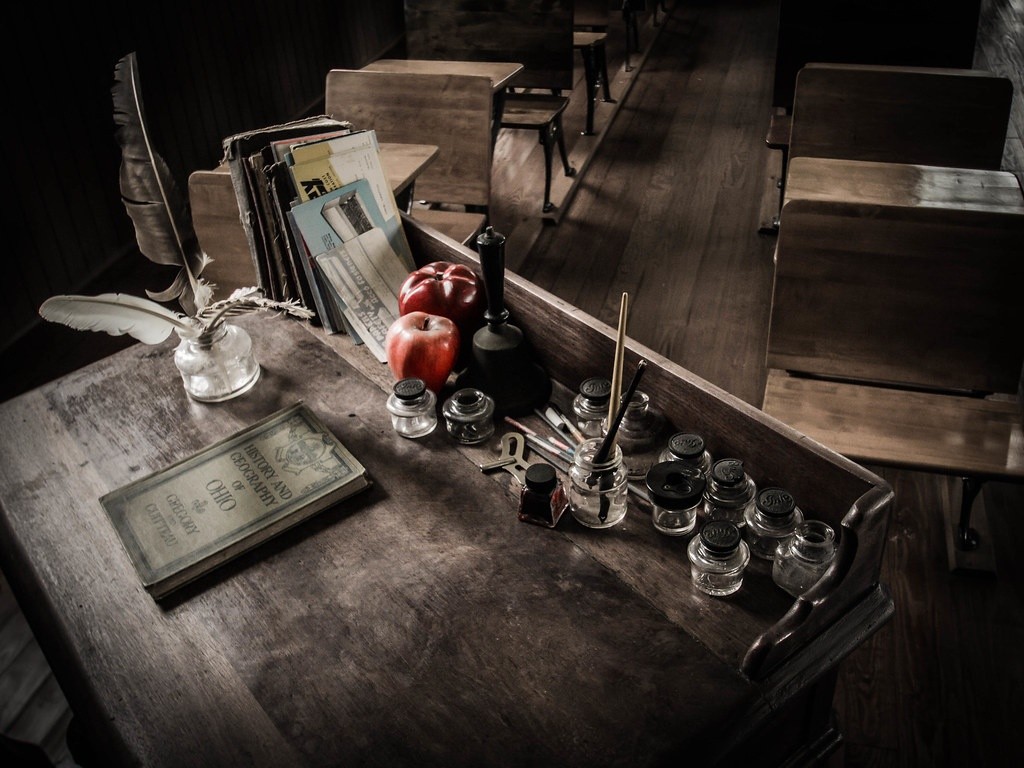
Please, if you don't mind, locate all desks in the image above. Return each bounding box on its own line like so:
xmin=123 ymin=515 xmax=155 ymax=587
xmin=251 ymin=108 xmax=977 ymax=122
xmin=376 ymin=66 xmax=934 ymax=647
xmin=211 ymin=141 xmax=439 ymax=216
xmin=803 ymin=62 xmax=995 ymax=77
xmin=0 ymin=215 xmax=896 ymax=767
xmin=780 ymin=156 xmax=1024 ymax=213
xmin=360 ymin=59 xmax=524 ymax=162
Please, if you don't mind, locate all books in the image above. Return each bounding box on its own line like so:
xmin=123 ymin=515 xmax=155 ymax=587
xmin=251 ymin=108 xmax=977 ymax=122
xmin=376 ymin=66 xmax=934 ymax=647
xmin=223 ymin=113 xmax=419 ymax=364
xmin=97 ymin=399 xmax=376 ymax=603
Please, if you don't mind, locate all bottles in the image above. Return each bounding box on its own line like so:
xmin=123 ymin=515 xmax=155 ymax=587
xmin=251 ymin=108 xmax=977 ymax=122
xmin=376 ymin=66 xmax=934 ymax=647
xmin=175 ymin=316 xmax=260 ymax=404
xmin=687 ymin=520 xmax=751 ymax=595
xmin=386 ymin=378 xmax=437 ymax=439
xmin=703 ymin=458 xmax=757 ymax=528
xmin=650 ymin=484 xmax=697 ymax=535
xmin=743 ymin=488 xmax=805 ymax=572
xmin=574 ymin=380 xmax=610 ymax=437
xmin=772 ymin=521 xmax=837 ymax=599
xmin=570 ymin=438 xmax=628 ymax=528
xmin=616 ymin=391 xmax=659 ymax=479
xmin=659 ymin=432 xmax=713 ymax=479
xmin=443 ymin=388 xmax=496 ymax=444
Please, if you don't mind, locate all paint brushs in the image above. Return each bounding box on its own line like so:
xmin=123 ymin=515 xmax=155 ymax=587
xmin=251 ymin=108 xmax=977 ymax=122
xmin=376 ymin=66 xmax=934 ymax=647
xmin=585 ymin=291 xmax=649 ymax=523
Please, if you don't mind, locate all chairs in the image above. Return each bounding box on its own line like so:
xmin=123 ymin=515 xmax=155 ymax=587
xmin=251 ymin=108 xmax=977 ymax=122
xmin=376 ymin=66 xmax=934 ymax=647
xmin=785 ymin=62 xmax=1014 ymax=172
xmin=325 ymin=70 xmax=495 ymax=247
xmin=188 ymin=170 xmax=258 ymax=301
xmin=759 ymin=0 xmax=980 ymax=186
xmin=760 ymin=196 xmax=1024 ymax=552
xmin=403 ymin=0 xmax=579 ymax=213
xmin=573 ymin=1 xmax=673 ymax=137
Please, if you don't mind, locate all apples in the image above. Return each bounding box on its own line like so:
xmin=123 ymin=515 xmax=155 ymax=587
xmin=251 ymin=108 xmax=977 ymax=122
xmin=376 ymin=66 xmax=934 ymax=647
xmin=398 ymin=262 xmax=480 ymax=337
xmin=385 ymin=311 xmax=460 ymax=393
